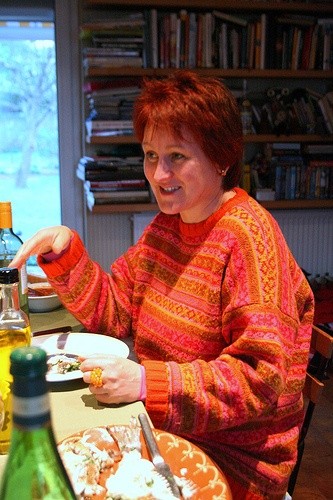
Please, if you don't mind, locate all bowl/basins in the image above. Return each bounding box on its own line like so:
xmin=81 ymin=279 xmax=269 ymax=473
xmin=27 ymin=281 xmax=62 ymax=312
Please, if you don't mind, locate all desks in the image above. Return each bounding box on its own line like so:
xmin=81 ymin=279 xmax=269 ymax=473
xmin=0 ymin=307 xmax=154 ymax=491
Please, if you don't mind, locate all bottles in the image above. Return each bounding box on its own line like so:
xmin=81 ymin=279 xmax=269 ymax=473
xmin=239 ymin=100 xmax=253 ymax=136
xmin=0 ymin=201 xmax=30 ymax=319
xmin=0 ymin=268 xmax=33 ymax=456
xmin=0 ymin=345 xmax=79 ymax=500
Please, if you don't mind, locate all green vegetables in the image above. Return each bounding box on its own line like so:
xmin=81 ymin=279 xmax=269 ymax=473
xmin=63 ymin=361 xmax=81 ymax=372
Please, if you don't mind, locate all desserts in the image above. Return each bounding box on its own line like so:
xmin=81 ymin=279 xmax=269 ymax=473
xmin=106 ymin=449 xmax=181 ymax=500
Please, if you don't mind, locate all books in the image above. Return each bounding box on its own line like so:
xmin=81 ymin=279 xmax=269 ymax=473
xmin=242 ymin=142 xmax=333 ymax=200
xmin=76 ymin=152 xmax=155 ymax=205
xmin=79 ymin=11 xmax=148 ymax=68
xmin=151 ymin=8 xmax=266 ymax=69
xmin=292 ymin=86 xmax=333 ymax=135
xmin=270 ymin=15 xmax=333 ymax=70
xmin=82 ymin=80 xmax=143 ymax=137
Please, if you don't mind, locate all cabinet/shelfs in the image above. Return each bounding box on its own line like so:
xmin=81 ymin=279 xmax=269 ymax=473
xmin=82 ymin=1 xmax=333 ymax=212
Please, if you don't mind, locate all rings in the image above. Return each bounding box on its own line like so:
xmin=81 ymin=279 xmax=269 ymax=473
xmin=90 ymin=367 xmax=102 ymax=389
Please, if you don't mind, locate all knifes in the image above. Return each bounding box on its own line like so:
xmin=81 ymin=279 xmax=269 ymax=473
xmin=138 ymin=413 xmax=181 ymax=499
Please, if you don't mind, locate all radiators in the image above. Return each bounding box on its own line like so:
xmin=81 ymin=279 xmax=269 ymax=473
xmin=81 ymin=211 xmax=332 ymax=281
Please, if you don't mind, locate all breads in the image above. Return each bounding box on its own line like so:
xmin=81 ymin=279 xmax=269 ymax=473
xmin=26 ymin=273 xmax=57 ymax=297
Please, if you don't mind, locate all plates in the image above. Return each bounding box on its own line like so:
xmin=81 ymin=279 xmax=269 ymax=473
xmin=29 ymin=332 xmax=130 ymax=383
xmin=56 ymin=425 xmax=233 ymax=500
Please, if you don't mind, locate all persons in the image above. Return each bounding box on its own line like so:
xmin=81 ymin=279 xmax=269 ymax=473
xmin=8 ymin=70 xmax=315 ymax=499
